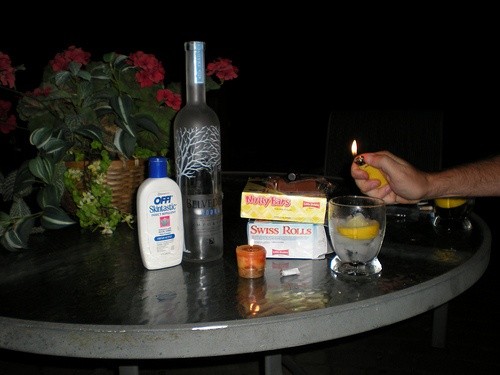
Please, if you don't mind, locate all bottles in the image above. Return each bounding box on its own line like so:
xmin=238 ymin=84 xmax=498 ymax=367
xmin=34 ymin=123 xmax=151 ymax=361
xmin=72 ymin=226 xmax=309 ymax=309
xmin=174 ymin=40 xmax=223 ymax=263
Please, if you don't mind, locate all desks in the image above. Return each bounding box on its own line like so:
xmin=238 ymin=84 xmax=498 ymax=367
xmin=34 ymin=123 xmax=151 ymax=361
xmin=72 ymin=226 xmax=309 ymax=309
xmin=0 ymin=212 xmax=492 ymax=375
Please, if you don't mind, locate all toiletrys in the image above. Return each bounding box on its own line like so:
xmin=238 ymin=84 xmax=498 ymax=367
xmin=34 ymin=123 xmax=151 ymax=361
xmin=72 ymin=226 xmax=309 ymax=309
xmin=136 ymin=156 xmax=184 ymax=270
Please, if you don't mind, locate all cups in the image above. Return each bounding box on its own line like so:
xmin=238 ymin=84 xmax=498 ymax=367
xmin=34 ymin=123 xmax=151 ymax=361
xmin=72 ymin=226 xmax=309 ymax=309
xmin=328 ymin=195 xmax=387 ymax=278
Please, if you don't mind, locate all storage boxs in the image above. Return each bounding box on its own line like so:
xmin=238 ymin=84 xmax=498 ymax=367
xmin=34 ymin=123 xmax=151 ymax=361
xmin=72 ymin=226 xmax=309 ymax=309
xmin=239 ymin=176 xmax=328 ymax=225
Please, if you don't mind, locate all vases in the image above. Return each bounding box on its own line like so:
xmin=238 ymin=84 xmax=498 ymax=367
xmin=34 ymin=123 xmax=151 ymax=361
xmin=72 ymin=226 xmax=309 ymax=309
xmin=60 ymin=159 xmax=145 ymax=218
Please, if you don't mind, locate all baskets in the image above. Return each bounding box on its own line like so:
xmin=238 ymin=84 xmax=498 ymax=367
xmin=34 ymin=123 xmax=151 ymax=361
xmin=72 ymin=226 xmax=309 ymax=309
xmin=55 ymin=159 xmax=146 ymax=223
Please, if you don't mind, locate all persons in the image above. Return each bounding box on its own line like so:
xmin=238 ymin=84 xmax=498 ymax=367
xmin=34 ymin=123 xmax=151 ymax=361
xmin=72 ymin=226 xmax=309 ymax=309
xmin=352 ymin=150 xmax=500 ymax=204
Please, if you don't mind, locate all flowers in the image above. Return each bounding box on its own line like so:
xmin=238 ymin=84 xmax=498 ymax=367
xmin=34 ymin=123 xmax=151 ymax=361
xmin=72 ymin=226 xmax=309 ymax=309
xmin=0 ymin=45 xmax=239 ymax=252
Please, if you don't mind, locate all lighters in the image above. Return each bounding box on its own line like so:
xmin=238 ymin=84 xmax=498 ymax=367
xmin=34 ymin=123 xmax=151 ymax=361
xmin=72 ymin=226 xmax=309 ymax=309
xmin=354 ymin=155 xmax=388 ymax=188
